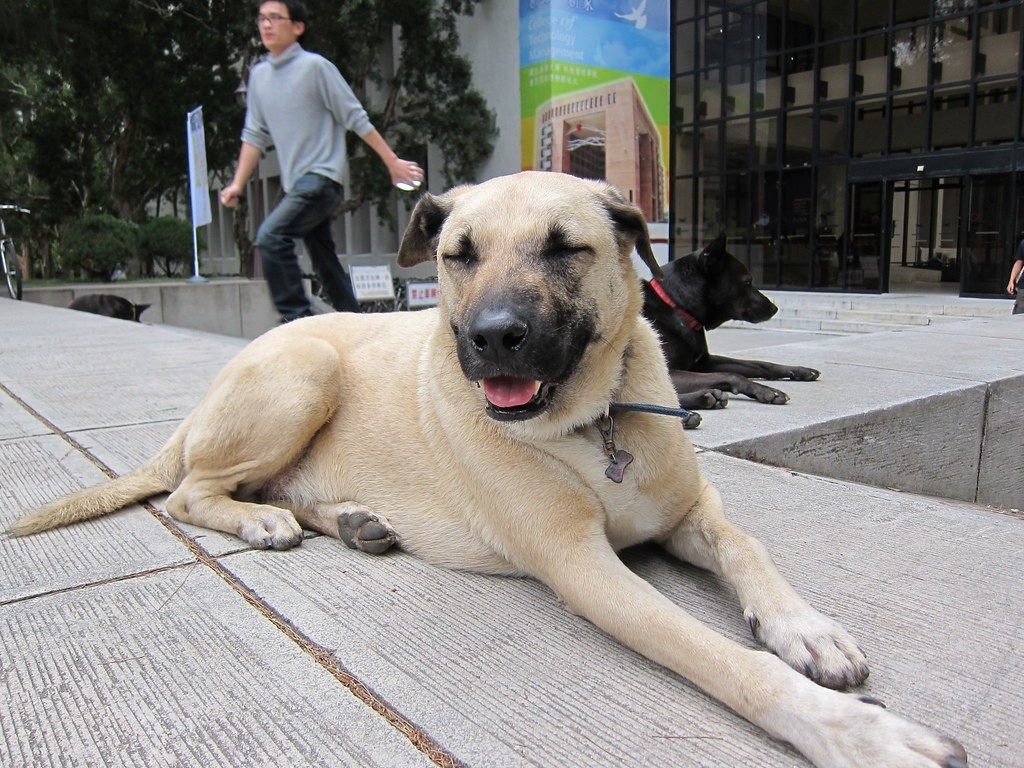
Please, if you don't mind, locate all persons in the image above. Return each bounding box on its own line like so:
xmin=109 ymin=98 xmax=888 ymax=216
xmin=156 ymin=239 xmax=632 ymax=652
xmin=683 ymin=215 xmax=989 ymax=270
xmin=815 ymin=213 xmax=836 ymax=287
xmin=220 ymin=0 xmax=426 ymax=326
xmin=1005 ymin=231 xmax=1024 ymax=316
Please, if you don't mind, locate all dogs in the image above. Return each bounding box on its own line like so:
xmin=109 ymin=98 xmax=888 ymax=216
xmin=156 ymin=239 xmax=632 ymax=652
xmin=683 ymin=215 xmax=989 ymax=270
xmin=639 ymin=229 xmax=821 ymax=410
xmin=68 ymin=294 xmax=152 ymax=323
xmin=2 ymin=171 xmax=970 ymax=768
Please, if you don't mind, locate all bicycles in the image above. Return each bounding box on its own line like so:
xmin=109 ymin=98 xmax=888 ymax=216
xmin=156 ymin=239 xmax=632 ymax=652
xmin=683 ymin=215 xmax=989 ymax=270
xmin=0 ymin=205 xmax=32 ymax=301
xmin=302 ymin=273 xmax=438 ymax=312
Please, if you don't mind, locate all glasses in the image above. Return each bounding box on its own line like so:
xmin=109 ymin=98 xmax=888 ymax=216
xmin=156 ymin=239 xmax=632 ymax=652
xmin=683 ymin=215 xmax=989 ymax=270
xmin=255 ymin=14 xmax=291 ymax=22
